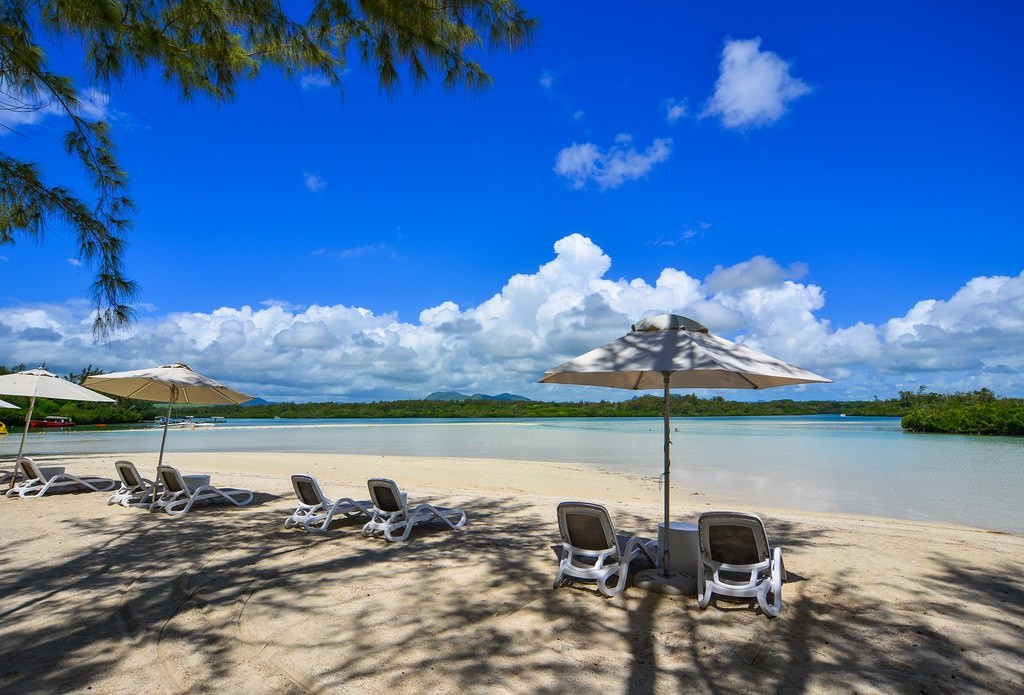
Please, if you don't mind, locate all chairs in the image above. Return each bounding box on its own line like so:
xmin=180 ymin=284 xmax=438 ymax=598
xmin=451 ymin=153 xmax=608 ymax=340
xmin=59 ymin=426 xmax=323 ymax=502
xmin=553 ymin=502 xmax=660 ymax=597
xmin=697 ymin=510 xmax=787 ymax=617
xmin=284 ymin=474 xmax=467 ymax=542
xmin=0 ymin=455 xmax=254 ymax=515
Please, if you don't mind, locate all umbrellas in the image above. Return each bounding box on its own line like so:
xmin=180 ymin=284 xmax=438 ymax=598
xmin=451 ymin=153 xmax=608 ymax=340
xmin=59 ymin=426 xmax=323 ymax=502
xmin=0 ymin=365 xmax=117 ymax=496
xmin=0 ymin=399 xmax=22 ymax=409
xmin=536 ymin=313 xmax=834 ymax=596
xmin=81 ymin=362 xmax=256 ymax=507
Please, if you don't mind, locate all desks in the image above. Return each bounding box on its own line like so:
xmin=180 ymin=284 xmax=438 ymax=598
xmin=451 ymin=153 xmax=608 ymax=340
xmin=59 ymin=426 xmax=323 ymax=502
xmin=39 ymin=467 xmax=66 ymax=491
xmin=181 ymin=474 xmax=210 ymax=490
xmin=658 ymin=522 xmax=698 ymax=571
xmin=400 ymin=493 xmax=407 ymax=510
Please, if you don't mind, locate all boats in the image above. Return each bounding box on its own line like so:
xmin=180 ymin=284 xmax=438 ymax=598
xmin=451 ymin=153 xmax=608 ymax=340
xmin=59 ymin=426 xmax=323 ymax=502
xmin=0 ymin=421 xmax=7 ymax=434
xmin=29 ymin=415 xmax=75 ymax=427
xmin=178 ymin=420 xmax=197 ymax=429
